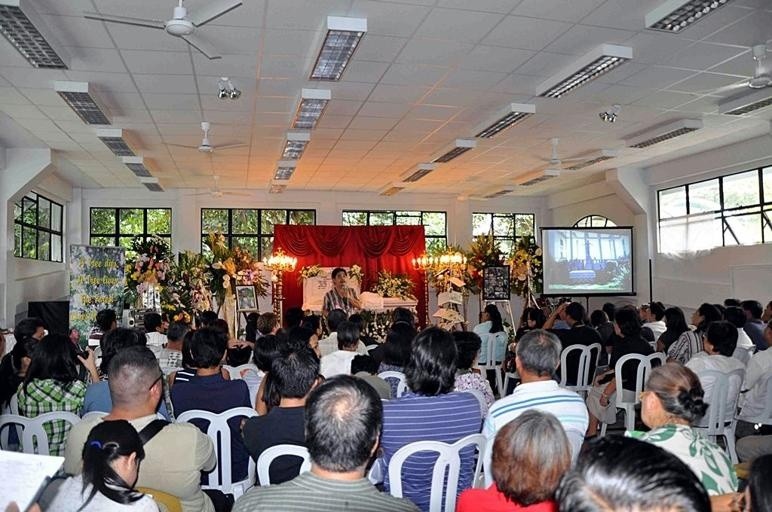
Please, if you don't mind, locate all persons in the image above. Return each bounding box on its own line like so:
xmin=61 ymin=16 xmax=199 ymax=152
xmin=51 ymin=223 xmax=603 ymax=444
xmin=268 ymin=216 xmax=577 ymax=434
xmin=240 ymin=290 xmax=255 ymax=309
xmin=323 ymin=267 xmax=361 ymax=315
xmin=1 ymin=298 xmax=770 ymax=512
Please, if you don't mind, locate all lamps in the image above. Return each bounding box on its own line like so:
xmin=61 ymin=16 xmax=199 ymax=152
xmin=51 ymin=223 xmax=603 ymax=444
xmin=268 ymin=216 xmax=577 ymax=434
xmin=0 ymin=1 xmax=166 ymax=194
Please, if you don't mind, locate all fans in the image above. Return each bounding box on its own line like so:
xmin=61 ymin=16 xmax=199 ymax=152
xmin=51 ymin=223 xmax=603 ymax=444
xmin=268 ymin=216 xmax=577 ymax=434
xmin=84 ymin=0 xmax=236 ymax=60
xmin=161 ymin=122 xmax=249 ymax=163
xmin=731 ymin=44 xmax=772 ymax=91
